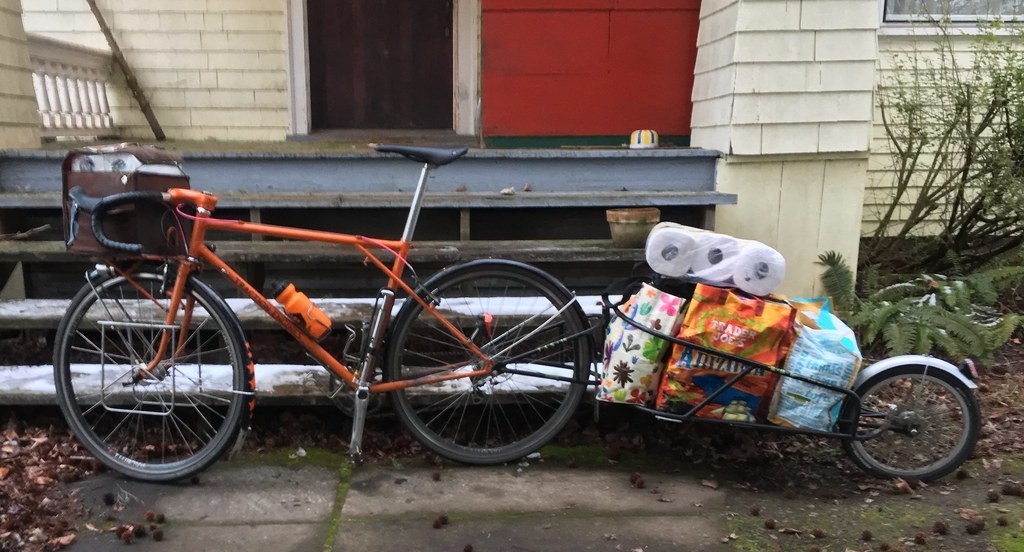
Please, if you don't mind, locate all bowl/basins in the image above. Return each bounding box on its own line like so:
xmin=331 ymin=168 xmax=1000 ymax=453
xmin=606 ymin=208 xmax=660 ymax=247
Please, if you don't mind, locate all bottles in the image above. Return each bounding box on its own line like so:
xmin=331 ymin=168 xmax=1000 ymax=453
xmin=271 ymin=278 xmax=332 ymax=339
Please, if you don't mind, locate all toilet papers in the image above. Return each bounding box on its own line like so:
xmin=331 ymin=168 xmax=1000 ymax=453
xmin=646 ymin=222 xmax=785 ymax=297
xmin=71 ymin=151 xmax=142 ymax=175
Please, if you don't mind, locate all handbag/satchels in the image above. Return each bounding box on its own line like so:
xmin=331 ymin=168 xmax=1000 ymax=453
xmin=769 ymin=294 xmax=862 ymax=432
xmin=596 ymin=280 xmax=690 ymax=407
xmin=655 ymin=287 xmax=797 ymax=423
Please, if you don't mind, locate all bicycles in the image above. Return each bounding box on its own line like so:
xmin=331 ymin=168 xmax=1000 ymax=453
xmin=52 ymin=144 xmax=606 ymax=481
